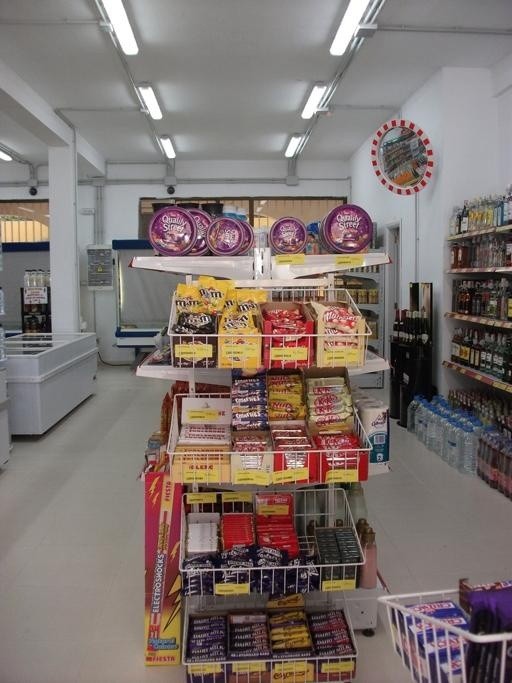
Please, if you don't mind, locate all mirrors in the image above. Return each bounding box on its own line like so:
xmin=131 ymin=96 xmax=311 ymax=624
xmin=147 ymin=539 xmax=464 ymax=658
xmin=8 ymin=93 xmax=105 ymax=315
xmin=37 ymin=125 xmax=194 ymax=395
xmin=370 ymin=118 xmax=433 ymax=195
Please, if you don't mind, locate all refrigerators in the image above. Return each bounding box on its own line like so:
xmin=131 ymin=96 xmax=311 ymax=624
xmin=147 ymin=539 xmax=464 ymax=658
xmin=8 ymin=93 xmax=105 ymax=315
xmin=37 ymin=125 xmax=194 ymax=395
xmin=20 ymin=287 xmax=51 ymax=332
xmin=3 ymin=332 xmax=99 ymax=435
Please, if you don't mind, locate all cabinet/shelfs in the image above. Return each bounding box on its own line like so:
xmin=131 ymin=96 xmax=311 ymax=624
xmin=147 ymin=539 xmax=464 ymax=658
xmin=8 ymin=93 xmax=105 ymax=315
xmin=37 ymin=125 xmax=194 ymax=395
xmin=128 ymin=247 xmax=393 ymax=636
xmin=442 ymin=225 xmax=512 ymax=393
xmin=383 ymin=131 xmax=425 ymax=174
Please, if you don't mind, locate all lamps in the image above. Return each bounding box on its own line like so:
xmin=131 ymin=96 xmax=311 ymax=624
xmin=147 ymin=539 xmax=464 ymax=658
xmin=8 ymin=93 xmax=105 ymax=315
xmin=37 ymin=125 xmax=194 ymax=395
xmin=285 ymin=134 xmax=302 ymax=158
xmin=329 ymin=0 xmax=371 ymax=56
xmin=159 ymin=135 xmax=176 ymax=158
xmin=101 ymin=1 xmax=139 ymax=56
xmin=301 ymin=81 xmax=328 ymax=119
xmin=137 ymin=81 xmax=163 ymax=120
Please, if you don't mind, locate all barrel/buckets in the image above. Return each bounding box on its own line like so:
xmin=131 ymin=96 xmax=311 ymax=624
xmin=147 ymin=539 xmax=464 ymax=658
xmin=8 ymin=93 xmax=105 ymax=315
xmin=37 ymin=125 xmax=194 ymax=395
xmin=406 ymin=394 xmax=497 ymax=474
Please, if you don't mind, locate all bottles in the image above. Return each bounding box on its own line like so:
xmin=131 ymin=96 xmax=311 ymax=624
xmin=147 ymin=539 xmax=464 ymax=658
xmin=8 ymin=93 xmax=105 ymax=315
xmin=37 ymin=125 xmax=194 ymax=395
xmin=24 ymin=268 xmax=51 ymax=286
xmin=449 ymin=183 xmax=511 ymax=236
xmin=450 ymin=236 xmax=512 ymax=268
xmin=405 ymin=389 xmax=512 ymax=498
xmin=393 ymin=308 xmax=430 ymax=345
xmin=450 ymin=327 xmax=510 ymax=385
xmin=450 ymin=277 xmax=511 ymax=322
xmin=295 ymin=481 xmax=378 ymax=589
